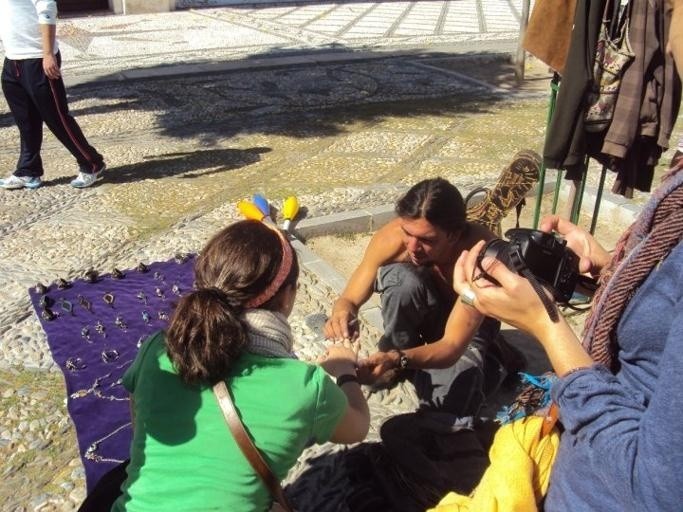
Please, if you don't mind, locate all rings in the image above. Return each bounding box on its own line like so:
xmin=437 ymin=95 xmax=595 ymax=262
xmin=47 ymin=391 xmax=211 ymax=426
xmin=461 ymin=286 xmax=474 ymax=307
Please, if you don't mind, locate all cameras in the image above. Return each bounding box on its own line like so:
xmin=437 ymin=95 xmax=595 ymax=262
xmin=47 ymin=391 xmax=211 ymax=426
xmin=476 ymin=227 xmax=581 ymax=306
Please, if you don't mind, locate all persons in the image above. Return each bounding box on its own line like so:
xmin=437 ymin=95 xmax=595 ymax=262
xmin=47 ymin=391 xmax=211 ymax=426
xmin=324 ymin=177 xmax=532 ymax=420
xmin=449 ymin=1 xmax=682 ymax=510
xmin=74 ymin=215 xmax=372 ymax=509
xmin=0 ymin=0 xmax=109 ymax=191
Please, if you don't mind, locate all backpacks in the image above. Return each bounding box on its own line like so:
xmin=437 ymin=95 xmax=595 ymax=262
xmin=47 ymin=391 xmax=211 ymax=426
xmin=381 ymin=414 xmax=490 ymax=505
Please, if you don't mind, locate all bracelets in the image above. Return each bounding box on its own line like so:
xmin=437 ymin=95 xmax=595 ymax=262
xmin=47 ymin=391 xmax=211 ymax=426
xmin=336 ymin=374 xmax=358 ymax=386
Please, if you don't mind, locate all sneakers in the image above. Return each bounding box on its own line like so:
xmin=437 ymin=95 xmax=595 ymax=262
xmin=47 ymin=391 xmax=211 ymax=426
xmin=432 ymin=410 xmax=472 ymax=435
xmin=364 ymin=367 xmax=400 ymax=392
xmin=0 ymin=172 xmax=42 ymax=188
xmin=71 ymin=166 xmax=106 ymax=188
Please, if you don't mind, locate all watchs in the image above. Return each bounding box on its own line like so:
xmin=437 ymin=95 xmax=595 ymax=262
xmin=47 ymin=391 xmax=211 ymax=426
xmin=389 ymin=347 xmax=410 ymax=382
